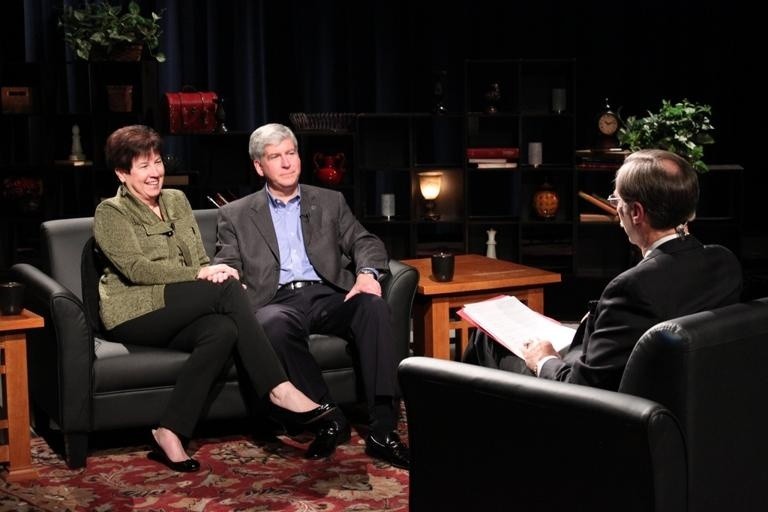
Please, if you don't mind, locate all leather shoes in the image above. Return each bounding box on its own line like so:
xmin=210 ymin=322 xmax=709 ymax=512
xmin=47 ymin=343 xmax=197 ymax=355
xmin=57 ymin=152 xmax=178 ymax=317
xmin=365 ymin=431 xmax=409 ymax=471
xmin=305 ymin=418 xmax=352 ymax=460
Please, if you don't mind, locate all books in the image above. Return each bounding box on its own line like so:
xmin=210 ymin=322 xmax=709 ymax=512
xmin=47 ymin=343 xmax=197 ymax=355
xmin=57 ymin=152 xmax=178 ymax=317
xmin=466 ymin=147 xmax=520 ymax=169
xmin=578 ymin=191 xmax=619 ymax=216
xmin=580 ymin=214 xmax=619 ymax=222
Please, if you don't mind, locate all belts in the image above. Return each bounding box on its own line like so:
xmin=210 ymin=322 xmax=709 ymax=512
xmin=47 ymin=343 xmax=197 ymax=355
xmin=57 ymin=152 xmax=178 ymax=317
xmin=277 ymin=280 xmax=319 ymax=293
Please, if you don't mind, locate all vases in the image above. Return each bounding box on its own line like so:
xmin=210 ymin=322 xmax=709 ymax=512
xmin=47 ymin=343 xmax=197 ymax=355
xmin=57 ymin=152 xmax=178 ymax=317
xmin=108 ymin=83 xmax=133 ymax=112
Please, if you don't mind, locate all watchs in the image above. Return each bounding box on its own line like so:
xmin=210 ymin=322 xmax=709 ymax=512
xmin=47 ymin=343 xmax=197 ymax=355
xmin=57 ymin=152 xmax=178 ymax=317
xmin=356 ymin=268 xmax=378 ymax=281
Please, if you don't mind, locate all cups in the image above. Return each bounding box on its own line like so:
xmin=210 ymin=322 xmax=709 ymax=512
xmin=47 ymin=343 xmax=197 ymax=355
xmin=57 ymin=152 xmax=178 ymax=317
xmin=429 ymin=251 xmax=457 ymax=284
xmin=1 ymin=282 xmax=26 ymax=315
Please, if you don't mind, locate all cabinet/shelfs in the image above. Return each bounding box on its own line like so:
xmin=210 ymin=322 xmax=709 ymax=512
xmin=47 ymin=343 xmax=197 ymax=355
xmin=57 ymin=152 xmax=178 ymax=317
xmin=577 ymin=165 xmax=745 ymax=278
xmin=463 ymin=61 xmax=577 ymax=281
xmin=290 ymin=112 xmax=468 ymax=257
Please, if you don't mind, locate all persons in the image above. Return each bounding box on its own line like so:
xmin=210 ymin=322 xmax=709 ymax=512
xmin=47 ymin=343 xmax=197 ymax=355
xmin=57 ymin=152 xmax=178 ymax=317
xmin=92 ymin=124 xmax=337 ymax=472
xmin=458 ymin=146 xmax=745 ymax=393
xmin=206 ymin=123 xmax=410 ymax=470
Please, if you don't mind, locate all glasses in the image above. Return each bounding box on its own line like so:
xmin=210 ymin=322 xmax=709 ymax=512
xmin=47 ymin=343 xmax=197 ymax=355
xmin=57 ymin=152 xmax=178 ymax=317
xmin=608 ymin=195 xmax=622 ymax=208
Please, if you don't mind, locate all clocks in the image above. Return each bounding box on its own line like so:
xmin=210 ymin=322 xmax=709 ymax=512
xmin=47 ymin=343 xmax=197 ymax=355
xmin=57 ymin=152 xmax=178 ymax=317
xmin=599 ymin=97 xmax=621 ymax=149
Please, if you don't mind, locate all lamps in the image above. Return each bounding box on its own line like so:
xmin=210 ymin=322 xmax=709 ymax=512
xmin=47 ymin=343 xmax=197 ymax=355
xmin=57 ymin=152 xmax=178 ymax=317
xmin=416 ymin=171 xmax=445 ymax=222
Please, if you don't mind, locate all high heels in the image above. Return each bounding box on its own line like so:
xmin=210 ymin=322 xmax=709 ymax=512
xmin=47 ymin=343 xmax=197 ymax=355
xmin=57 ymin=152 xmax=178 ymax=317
xmin=149 ymin=429 xmax=201 ymax=472
xmin=265 ymin=401 xmax=337 ymax=433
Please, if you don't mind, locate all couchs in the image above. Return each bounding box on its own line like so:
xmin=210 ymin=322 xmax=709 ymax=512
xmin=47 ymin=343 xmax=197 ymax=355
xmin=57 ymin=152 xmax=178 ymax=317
xmin=10 ymin=208 xmax=420 ymax=467
xmin=396 ymin=297 xmax=767 ymax=511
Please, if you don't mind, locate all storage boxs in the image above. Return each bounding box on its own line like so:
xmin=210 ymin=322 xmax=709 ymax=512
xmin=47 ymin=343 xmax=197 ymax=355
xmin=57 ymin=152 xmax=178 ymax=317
xmin=4 ymin=178 xmax=43 ymax=224
xmin=1 ymin=86 xmax=35 ymax=114
xmin=165 ymin=93 xmax=220 ymax=133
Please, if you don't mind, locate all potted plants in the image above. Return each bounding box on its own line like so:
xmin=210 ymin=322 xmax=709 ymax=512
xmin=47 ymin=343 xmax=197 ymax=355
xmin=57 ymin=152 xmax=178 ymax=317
xmin=57 ymin=3 xmax=167 ymax=64
xmin=615 ymin=98 xmax=717 ymax=176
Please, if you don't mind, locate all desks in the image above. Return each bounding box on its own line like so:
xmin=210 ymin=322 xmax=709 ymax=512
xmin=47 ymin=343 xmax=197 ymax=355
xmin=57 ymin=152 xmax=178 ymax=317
xmin=0 ymin=307 xmax=46 ymax=478
xmin=394 ymin=254 xmax=562 ymax=362
